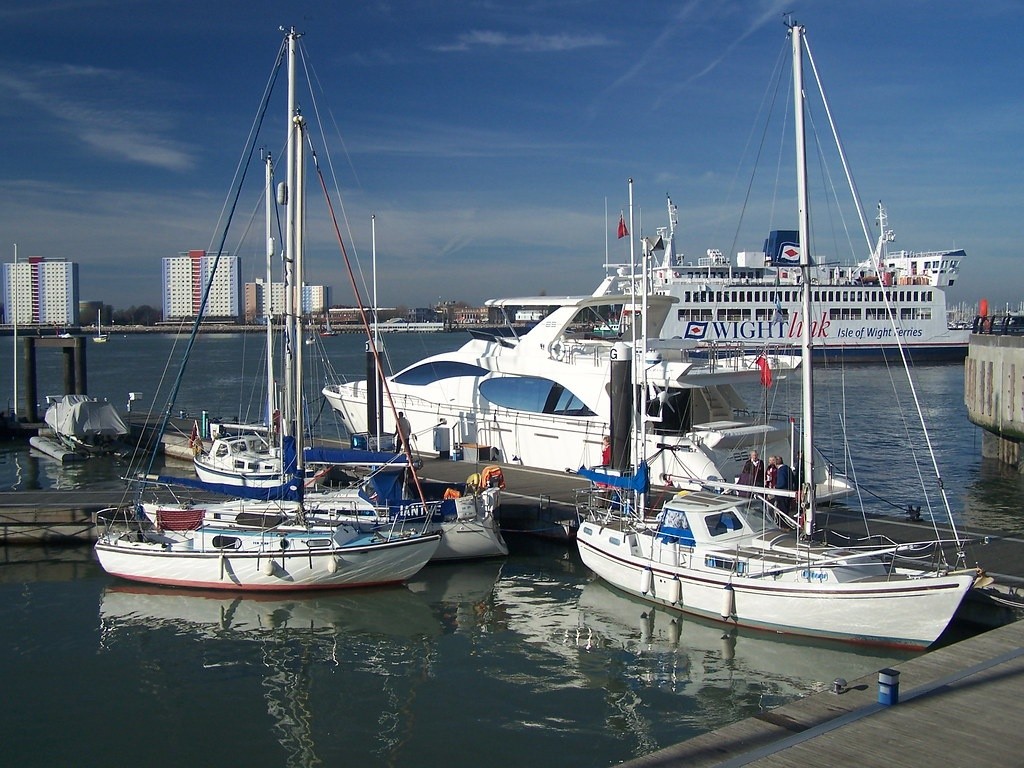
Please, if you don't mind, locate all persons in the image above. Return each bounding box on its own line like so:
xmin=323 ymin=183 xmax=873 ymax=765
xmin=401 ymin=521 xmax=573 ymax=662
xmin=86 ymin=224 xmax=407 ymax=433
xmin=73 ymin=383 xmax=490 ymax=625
xmin=393 ymin=411 xmax=413 ymax=458
xmin=599 ymin=436 xmax=611 ymax=509
xmin=737 ymin=450 xmax=795 ymax=533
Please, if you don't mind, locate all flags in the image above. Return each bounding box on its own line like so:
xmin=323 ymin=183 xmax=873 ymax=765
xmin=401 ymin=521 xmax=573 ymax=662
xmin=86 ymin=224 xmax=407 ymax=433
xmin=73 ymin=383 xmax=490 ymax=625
xmin=754 ymin=349 xmax=775 ymax=392
xmin=617 ymin=211 xmax=629 ymax=240
xmin=188 ymin=423 xmax=199 ymax=448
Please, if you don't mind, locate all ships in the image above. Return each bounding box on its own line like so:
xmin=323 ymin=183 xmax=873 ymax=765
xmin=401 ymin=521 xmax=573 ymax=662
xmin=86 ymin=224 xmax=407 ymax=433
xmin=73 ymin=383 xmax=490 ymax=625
xmin=586 ymin=191 xmax=970 ymax=365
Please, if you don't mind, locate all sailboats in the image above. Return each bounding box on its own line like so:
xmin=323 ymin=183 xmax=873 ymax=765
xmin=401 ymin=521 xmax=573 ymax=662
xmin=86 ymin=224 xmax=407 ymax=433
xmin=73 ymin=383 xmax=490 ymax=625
xmin=92 ymin=308 xmax=107 ymax=343
xmin=573 ymin=11 xmax=986 ymax=652
xmin=96 ymin=23 xmax=516 ymax=591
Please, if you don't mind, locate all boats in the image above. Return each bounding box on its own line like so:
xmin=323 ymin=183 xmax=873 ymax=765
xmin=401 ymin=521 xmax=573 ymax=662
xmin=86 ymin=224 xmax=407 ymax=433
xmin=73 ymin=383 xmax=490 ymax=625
xmin=44 ymin=393 xmax=131 ymax=454
xmin=305 ymin=336 xmax=316 ymax=345
xmin=61 ymin=333 xmax=73 ymax=339
xmin=319 ymin=288 xmax=856 ymax=507
